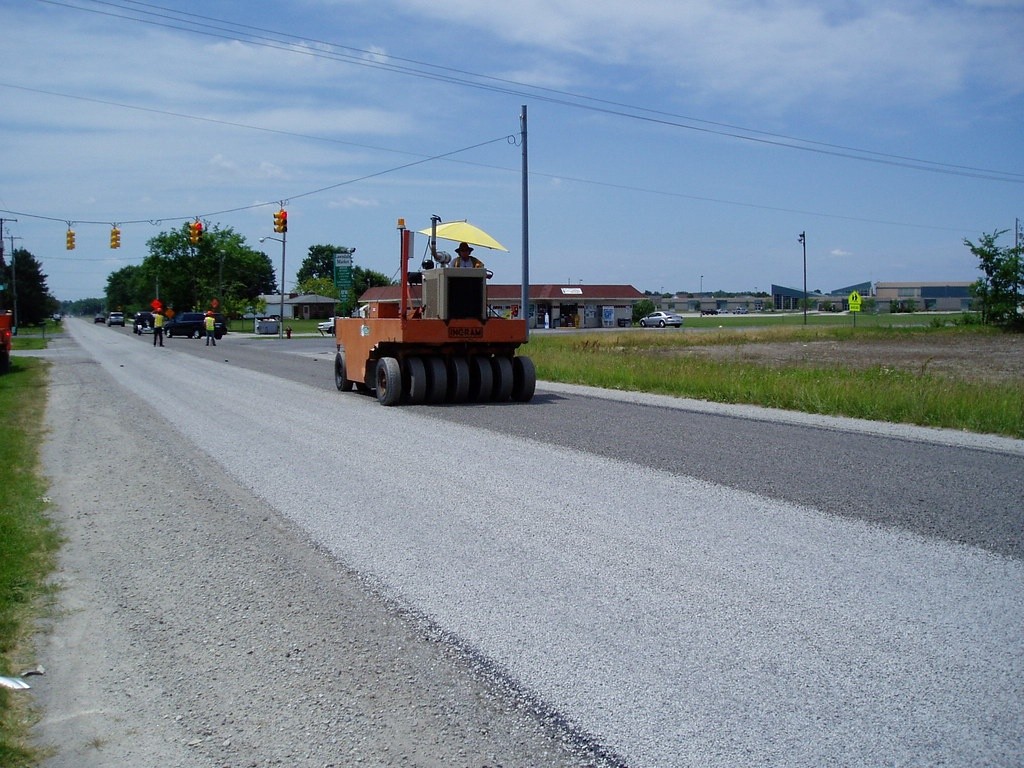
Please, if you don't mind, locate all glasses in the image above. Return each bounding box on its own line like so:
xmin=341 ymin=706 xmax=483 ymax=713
xmin=461 ymin=249 xmax=469 ymax=252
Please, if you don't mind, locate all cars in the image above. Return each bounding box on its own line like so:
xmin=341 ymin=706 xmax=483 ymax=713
xmin=639 ymin=311 xmax=683 ymax=328
xmin=701 ymin=307 xmax=748 ymax=316
xmin=53 ymin=314 xmax=61 ymax=322
xmin=318 ymin=317 xmax=349 ymax=333
xmin=94 ymin=313 xmax=105 ymax=323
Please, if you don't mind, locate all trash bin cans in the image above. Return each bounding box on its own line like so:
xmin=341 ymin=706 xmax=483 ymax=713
xmin=255 ymin=318 xmax=279 ymax=335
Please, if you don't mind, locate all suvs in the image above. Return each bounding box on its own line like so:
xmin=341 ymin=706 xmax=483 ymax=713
xmin=133 ymin=312 xmax=156 ymax=333
xmin=107 ymin=312 xmax=125 ymax=327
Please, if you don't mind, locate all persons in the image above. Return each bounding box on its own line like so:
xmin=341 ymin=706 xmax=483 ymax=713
xmin=449 ymin=242 xmax=484 ymax=268
xmin=203 ymin=311 xmax=217 ymax=346
xmin=151 ymin=308 xmax=165 ymax=347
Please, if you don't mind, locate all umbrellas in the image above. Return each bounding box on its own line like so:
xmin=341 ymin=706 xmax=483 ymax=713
xmin=416 ymin=219 xmax=511 ymax=252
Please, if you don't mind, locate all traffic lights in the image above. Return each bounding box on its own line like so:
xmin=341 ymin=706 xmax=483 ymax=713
xmin=273 ymin=212 xmax=280 ymax=232
xmin=280 ymin=211 xmax=287 ymax=232
xmin=116 ymin=229 xmax=121 ymax=249
xmin=70 ymin=231 xmax=76 ymax=250
xmin=195 ymin=222 xmax=203 ymax=244
xmin=188 ymin=223 xmax=194 ymax=243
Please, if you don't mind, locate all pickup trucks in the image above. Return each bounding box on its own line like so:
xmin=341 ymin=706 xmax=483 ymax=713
xmin=164 ymin=313 xmax=226 ymax=339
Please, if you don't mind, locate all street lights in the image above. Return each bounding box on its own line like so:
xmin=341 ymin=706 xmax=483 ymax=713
xmin=259 ymin=234 xmax=286 ymax=339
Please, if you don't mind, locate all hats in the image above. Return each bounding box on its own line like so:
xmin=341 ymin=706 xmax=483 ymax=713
xmin=455 ymin=243 xmax=473 ymax=252
xmin=157 ymin=307 xmax=162 ymax=312
xmin=208 ymin=311 xmax=212 ymax=316
